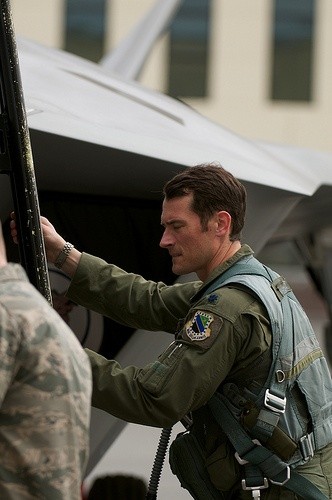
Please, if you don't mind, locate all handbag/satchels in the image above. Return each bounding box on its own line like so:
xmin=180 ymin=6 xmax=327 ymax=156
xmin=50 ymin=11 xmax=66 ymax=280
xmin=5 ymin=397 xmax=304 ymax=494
xmin=168 ymin=428 xmax=235 ymax=498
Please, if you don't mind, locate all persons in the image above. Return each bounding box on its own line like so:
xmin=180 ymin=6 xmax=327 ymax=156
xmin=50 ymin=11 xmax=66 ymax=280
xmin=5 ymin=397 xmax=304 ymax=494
xmin=11 ymin=164 xmax=332 ymax=500
xmin=0 ymin=227 xmax=94 ymax=500
xmin=88 ymin=471 xmax=148 ymax=500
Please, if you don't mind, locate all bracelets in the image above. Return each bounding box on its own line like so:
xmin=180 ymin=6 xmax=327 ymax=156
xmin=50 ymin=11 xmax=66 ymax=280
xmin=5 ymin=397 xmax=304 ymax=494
xmin=53 ymin=242 xmax=74 ymax=268
